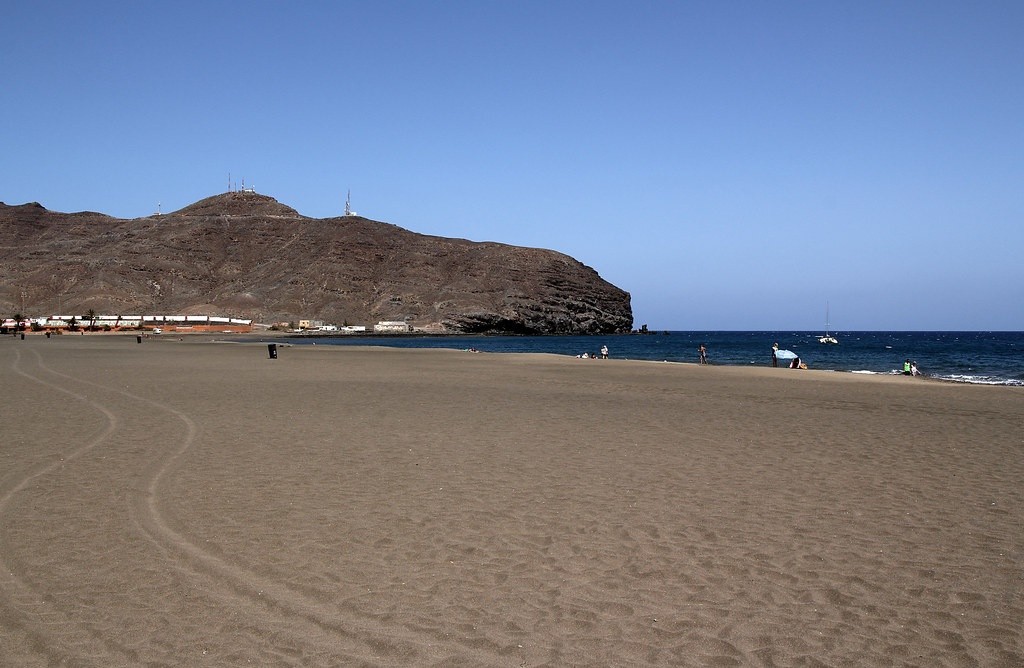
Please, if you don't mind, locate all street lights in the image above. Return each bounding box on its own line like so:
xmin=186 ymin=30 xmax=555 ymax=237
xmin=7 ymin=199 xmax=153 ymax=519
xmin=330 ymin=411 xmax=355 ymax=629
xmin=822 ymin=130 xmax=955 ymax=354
xmin=20 ymin=291 xmax=28 ymax=316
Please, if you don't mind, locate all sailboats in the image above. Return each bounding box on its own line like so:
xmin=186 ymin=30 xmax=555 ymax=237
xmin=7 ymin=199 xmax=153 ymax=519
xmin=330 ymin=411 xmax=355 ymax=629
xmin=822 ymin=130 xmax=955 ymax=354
xmin=817 ymin=299 xmax=838 ymax=345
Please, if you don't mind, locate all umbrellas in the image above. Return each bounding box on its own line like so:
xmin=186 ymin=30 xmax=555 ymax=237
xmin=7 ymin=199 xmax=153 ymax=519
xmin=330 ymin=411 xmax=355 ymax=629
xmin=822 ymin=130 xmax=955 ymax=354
xmin=774 ymin=349 xmax=798 ymax=359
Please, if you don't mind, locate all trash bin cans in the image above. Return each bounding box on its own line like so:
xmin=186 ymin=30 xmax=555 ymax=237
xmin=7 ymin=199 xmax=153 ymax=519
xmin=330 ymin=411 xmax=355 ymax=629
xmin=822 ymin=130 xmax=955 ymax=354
xmin=46 ymin=333 xmax=51 ymax=338
xmin=268 ymin=344 xmax=277 ymax=359
xmin=137 ymin=336 xmax=142 ymax=343
xmin=21 ymin=334 xmax=24 ymax=340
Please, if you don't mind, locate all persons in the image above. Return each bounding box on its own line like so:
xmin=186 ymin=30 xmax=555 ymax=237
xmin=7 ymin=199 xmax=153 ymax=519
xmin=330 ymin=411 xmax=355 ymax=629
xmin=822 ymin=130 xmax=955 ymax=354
xmin=772 ymin=343 xmax=778 ymax=366
xmin=601 ymin=345 xmax=608 ymax=358
xmin=903 ymin=358 xmax=911 ymax=376
xmin=698 ymin=344 xmax=708 ymax=365
xmin=911 ymin=361 xmax=917 ymax=376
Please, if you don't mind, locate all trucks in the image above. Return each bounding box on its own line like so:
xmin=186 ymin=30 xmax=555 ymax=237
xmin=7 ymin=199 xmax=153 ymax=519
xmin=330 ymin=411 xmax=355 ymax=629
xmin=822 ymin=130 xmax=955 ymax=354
xmin=153 ymin=328 xmax=161 ymax=334
